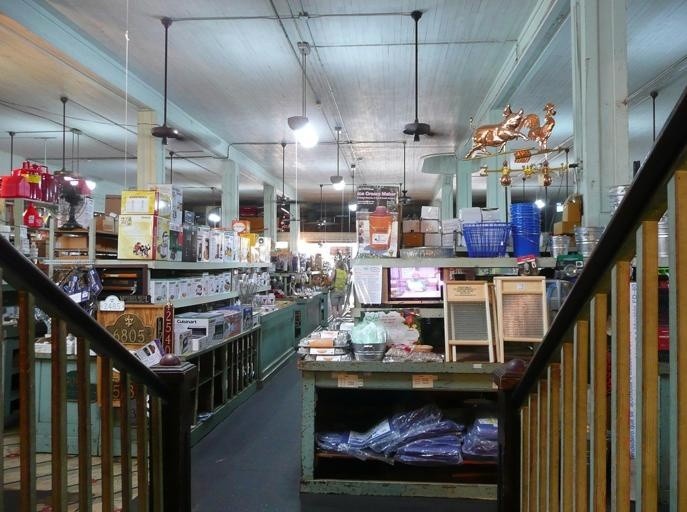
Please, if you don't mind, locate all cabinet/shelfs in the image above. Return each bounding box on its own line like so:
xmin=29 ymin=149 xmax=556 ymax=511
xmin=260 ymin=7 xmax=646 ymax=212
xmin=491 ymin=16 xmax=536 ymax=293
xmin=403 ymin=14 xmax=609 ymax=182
xmin=297 ymin=257 xmax=558 ymax=501
xmin=0 ymin=198 xmax=118 ymax=282
xmin=34 ymin=259 xmax=258 ymax=459
xmin=258 ymin=289 xmax=338 ymax=385
xmin=0 ymin=320 xmax=46 ymax=429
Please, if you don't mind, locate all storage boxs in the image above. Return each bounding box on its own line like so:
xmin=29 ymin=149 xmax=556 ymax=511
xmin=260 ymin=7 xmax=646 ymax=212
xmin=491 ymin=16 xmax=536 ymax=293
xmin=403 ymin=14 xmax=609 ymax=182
xmin=356 ymin=184 xmax=499 ymax=257
xmin=61 ymin=184 xmax=272 ymax=355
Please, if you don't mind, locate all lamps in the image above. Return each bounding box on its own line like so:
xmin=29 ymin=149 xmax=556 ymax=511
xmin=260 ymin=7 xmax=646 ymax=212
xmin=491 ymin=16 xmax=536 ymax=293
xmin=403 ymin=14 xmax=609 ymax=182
xmin=348 ymin=165 xmax=357 ymax=212
xmin=63 ymin=128 xmax=96 ymax=191
xmin=288 ymin=42 xmax=319 ymax=149
xmin=330 ymin=127 xmax=346 ymax=192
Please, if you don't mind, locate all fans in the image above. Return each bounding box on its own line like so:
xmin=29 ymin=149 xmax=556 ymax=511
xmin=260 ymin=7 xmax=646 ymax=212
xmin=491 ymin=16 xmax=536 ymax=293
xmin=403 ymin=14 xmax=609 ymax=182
xmin=50 ymin=189 xmax=88 ymax=230
xmin=10 ymin=16 xmax=222 ymax=182
xmin=399 ymin=10 xmax=472 ymax=142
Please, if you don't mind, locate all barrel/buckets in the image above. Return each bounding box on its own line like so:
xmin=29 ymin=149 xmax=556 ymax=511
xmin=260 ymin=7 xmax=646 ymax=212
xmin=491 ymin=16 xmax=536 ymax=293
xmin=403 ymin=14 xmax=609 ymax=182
xmin=573 ymin=224 xmax=605 ymax=258
xmin=351 ymin=342 xmax=387 ymax=360
xmin=549 ymin=235 xmax=569 ymax=258
xmin=510 ymin=203 xmax=541 ymax=257
xmin=658 ymin=222 xmax=670 ymax=268
xmin=609 ymin=184 xmax=629 ymax=216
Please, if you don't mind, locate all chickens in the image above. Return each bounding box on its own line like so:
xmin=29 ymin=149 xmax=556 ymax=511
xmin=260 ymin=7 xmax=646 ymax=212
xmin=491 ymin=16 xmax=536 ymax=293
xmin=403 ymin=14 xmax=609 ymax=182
xmin=516 ymin=103 xmax=557 ymax=150
